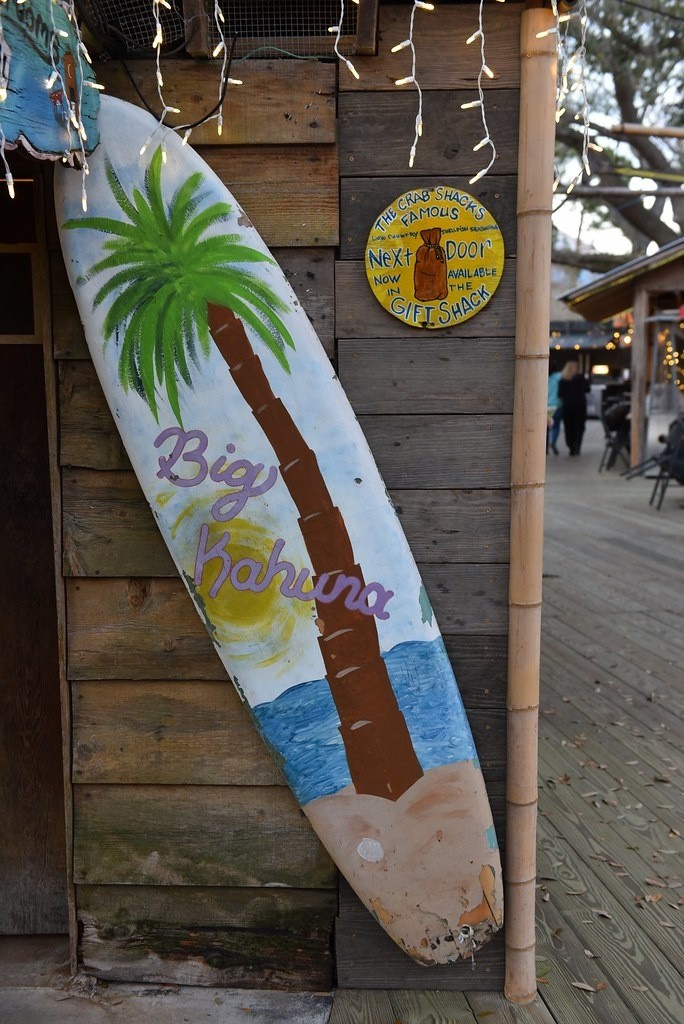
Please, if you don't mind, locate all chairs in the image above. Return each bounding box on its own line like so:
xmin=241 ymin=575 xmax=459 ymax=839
xmin=598 ymin=408 xmax=631 ymax=471
xmin=620 ymin=427 xmax=684 ymax=511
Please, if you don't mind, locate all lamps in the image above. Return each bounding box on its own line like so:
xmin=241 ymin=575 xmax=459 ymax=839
xmin=0 ymin=0 xmax=596 ymax=216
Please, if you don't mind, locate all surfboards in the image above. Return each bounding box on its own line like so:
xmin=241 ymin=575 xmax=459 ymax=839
xmin=55 ymin=94 xmax=504 ymax=969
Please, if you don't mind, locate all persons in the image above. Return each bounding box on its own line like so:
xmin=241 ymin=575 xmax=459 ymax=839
xmin=548 ymin=372 xmax=564 ymax=455
xmin=599 ymin=366 xmax=631 ymax=457
xmin=556 ymin=360 xmax=592 ymax=459
